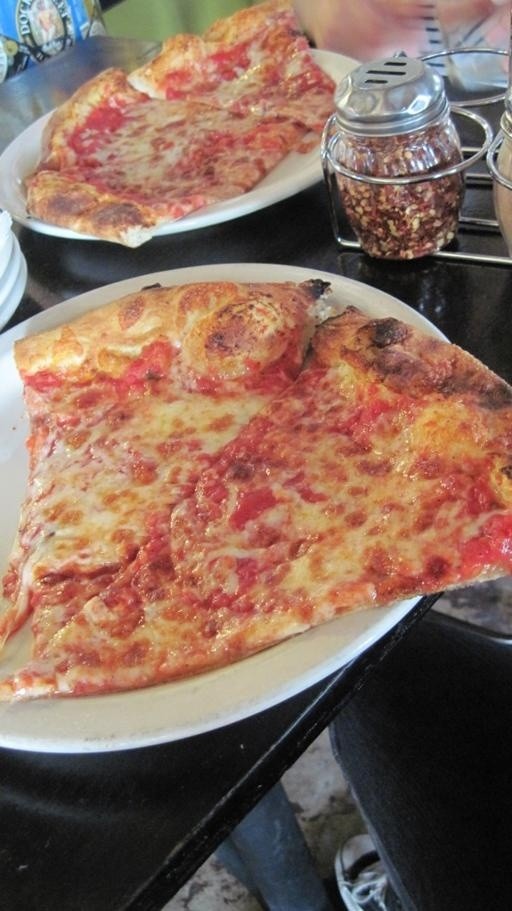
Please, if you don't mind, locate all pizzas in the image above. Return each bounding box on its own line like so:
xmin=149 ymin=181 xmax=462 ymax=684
xmin=21 ymin=0 xmax=337 ymax=249
xmin=0 ymin=279 xmax=512 ymax=703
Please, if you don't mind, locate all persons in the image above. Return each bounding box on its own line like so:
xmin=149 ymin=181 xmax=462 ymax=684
xmin=329 ymin=610 xmax=510 ymax=911
xmin=291 ymin=0 xmax=512 ymax=90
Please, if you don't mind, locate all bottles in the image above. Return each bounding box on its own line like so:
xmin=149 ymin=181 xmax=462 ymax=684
xmin=331 ymin=55 xmax=469 ymax=267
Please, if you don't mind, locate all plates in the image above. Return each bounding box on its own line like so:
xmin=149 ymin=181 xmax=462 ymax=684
xmin=1 ymin=47 xmax=366 ymax=243
xmin=2 ymin=260 xmax=453 ymax=758
